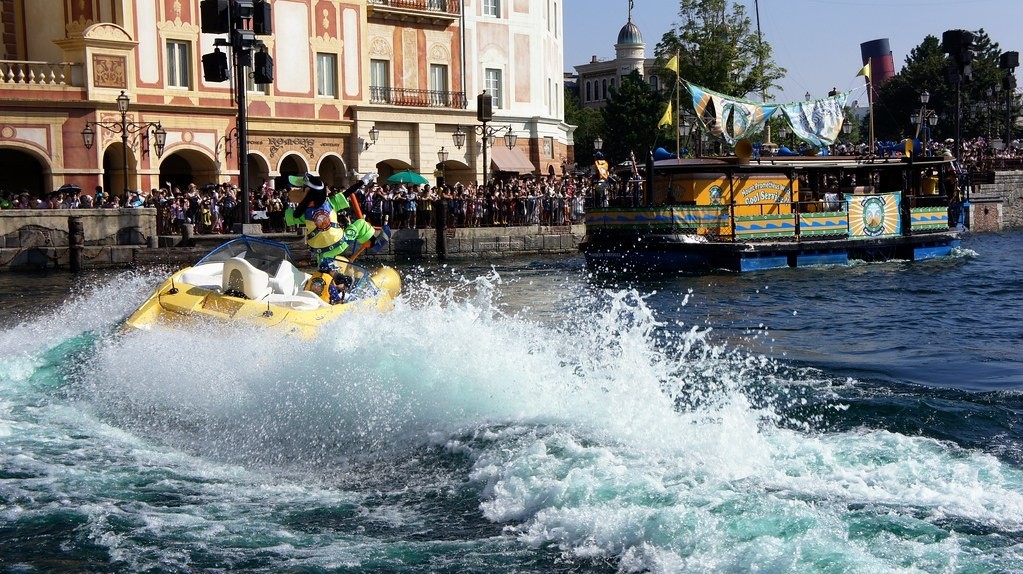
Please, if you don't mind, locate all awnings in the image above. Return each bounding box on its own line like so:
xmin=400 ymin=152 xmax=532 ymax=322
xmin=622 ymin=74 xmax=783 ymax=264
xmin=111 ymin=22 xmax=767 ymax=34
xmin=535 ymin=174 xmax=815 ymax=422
xmin=491 ymin=146 xmax=536 ymax=171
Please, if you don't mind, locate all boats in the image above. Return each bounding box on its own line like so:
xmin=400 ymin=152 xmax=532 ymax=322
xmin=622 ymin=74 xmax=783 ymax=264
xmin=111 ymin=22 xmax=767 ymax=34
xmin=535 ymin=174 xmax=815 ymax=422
xmin=116 ymin=234 xmax=404 ymax=353
xmin=577 ymin=153 xmax=969 ymax=276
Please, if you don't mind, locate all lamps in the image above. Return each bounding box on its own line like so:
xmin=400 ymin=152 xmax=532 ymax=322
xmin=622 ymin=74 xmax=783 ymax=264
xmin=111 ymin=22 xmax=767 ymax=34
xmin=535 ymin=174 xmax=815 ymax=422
xmin=365 ymin=124 xmax=381 ymax=151
xmin=487 ymin=131 xmax=496 ymax=149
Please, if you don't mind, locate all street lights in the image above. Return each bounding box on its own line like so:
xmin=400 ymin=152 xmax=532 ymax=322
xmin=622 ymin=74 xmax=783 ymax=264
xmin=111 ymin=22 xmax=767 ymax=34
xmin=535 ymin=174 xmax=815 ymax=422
xmin=911 ymin=88 xmax=939 ymax=157
xmin=200 ymin=0 xmax=274 ymax=224
xmin=778 ymin=125 xmax=796 ymax=151
xmin=995 ymin=81 xmax=1001 ymax=138
xmin=453 ymin=90 xmax=518 ymax=224
xmin=80 ymin=88 xmax=167 ymax=208
xmin=986 ymin=87 xmax=993 ymax=152
xmin=843 ymin=118 xmax=853 ymax=151
xmin=437 ymin=146 xmax=449 ymax=187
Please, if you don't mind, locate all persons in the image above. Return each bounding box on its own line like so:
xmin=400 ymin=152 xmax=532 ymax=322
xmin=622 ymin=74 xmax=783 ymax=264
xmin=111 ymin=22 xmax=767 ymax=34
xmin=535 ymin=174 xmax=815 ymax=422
xmin=799 ymin=135 xmax=1023 ymax=210
xmin=302 ymin=258 xmax=347 ymax=306
xmin=0 ymin=152 xmax=644 ymax=236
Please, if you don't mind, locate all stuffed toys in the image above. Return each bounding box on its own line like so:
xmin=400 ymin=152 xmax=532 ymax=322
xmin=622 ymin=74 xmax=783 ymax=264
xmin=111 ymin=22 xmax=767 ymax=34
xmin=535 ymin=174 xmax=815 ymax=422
xmin=286 ymin=172 xmax=391 ymax=270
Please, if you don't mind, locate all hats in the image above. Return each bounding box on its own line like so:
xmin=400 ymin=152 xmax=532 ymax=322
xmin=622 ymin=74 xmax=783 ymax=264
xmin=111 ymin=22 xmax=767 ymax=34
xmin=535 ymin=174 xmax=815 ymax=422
xmin=136 ymin=189 xmax=143 ymax=194
xmin=103 ymin=192 xmax=110 ymax=197
xmin=96 ymin=193 xmax=103 ymax=198
xmin=231 ymin=184 xmax=238 ymax=189
xmin=273 ymin=190 xmax=280 ymax=195
xmin=188 ymin=183 xmax=196 ymax=190
xmin=319 ymin=259 xmax=340 ymax=273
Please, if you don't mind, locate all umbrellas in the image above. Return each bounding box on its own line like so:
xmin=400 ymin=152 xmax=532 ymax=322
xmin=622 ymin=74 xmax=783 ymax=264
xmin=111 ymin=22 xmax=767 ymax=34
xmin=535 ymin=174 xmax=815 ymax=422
xmin=521 ymin=173 xmax=536 ymax=180
xmin=195 ymin=183 xmax=215 ymax=189
xmin=387 ymin=169 xmax=429 ymax=185
xmin=58 ymin=183 xmax=81 ymax=192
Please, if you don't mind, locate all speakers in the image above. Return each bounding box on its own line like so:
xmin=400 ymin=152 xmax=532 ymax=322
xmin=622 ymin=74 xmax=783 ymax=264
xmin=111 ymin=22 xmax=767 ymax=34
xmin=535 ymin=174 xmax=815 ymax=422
xmin=202 ymin=52 xmax=228 ymax=82
xmin=200 ymin=0 xmax=229 ymax=34
xmin=254 ymin=51 xmax=273 ymax=84
xmin=253 ymin=2 xmax=271 ymax=35
